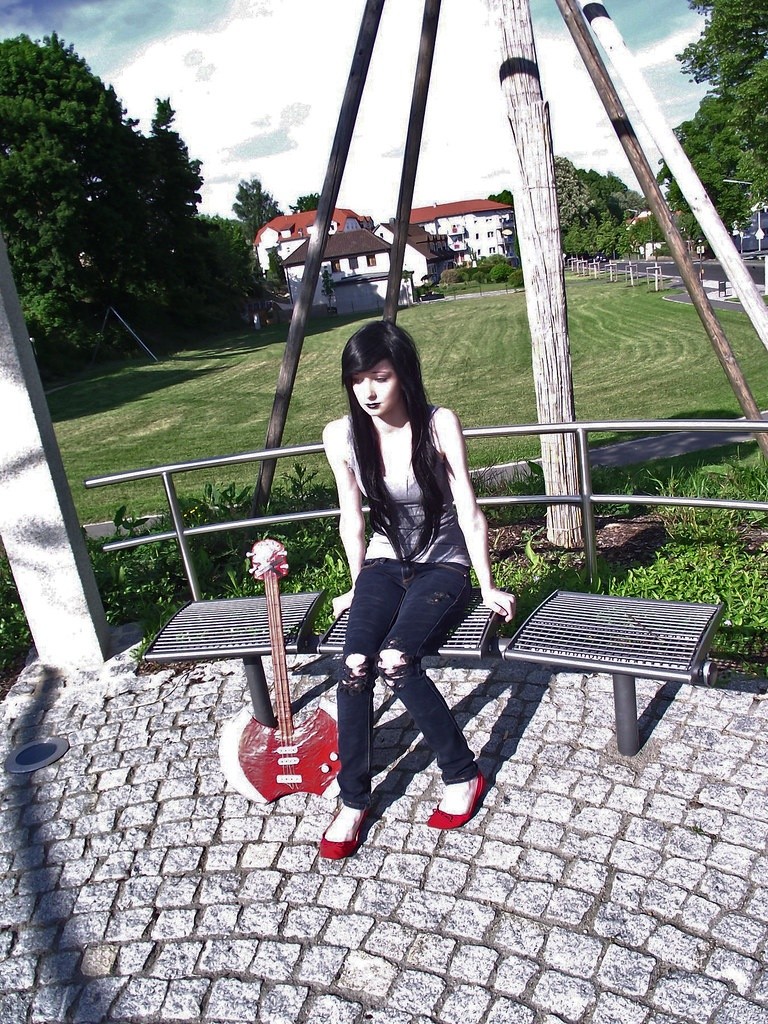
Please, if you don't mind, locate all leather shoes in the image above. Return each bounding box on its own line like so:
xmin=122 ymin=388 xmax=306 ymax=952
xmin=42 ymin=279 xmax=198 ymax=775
xmin=320 ymin=805 xmax=368 ymax=860
xmin=427 ymin=768 xmax=488 ymax=830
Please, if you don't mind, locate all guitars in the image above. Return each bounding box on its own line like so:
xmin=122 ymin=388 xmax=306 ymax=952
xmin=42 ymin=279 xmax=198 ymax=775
xmin=212 ymin=531 xmax=346 ymax=812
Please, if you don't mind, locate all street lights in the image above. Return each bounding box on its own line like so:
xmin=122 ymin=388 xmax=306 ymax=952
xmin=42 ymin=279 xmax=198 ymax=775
xmin=722 ymin=178 xmax=762 ymax=262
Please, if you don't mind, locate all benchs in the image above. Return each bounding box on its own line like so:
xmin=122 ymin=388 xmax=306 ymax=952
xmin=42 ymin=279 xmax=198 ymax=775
xmin=142 ymin=587 xmax=727 ymax=756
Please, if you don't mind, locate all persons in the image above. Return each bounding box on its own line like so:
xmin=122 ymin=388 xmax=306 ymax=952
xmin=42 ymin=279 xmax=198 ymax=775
xmin=317 ymin=321 xmax=515 ymax=861
